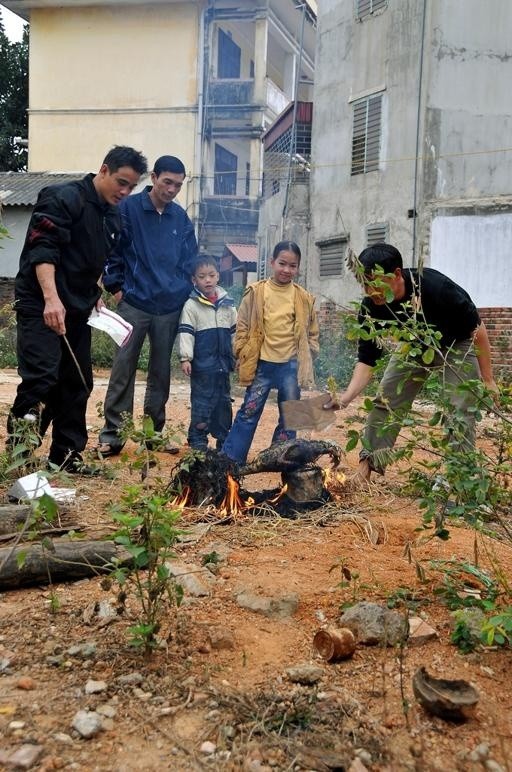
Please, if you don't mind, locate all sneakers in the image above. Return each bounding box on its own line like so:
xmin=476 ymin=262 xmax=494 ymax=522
xmin=141 ymin=437 xmax=180 ymax=454
xmin=45 ymin=455 xmax=100 ymax=477
xmin=89 ymin=441 xmax=125 ymax=456
xmin=7 ymin=451 xmax=40 ymax=468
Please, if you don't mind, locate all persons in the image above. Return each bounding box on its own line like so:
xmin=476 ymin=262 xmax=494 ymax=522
xmin=5 ymin=141 xmax=151 ymax=477
xmin=210 ymin=239 xmax=323 ymax=469
xmin=85 ymin=154 xmax=202 ymax=462
xmin=321 ymin=240 xmax=504 ymax=523
xmin=172 ymin=251 xmax=241 ymax=456
